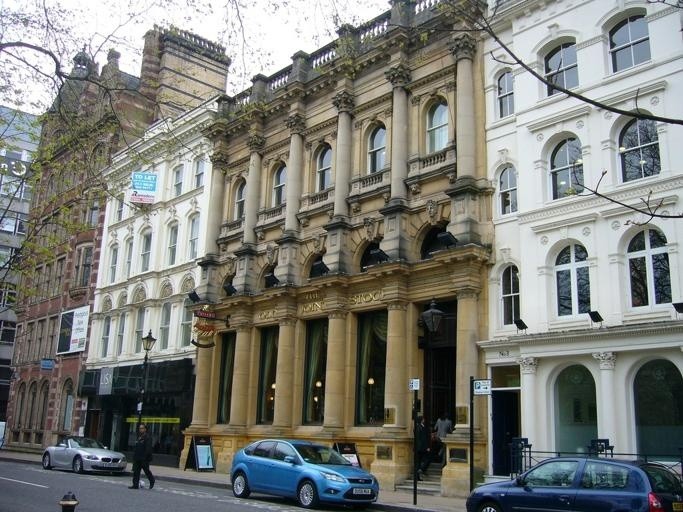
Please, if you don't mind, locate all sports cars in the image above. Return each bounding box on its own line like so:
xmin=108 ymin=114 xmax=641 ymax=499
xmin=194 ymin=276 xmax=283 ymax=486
xmin=40 ymin=436 xmax=127 ymax=473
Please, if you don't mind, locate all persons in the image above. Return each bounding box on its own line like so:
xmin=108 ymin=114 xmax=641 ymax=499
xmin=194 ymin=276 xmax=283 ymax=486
xmin=434 ymin=413 xmax=453 ymax=466
xmin=414 ymin=416 xmax=432 ymax=481
xmin=128 ymin=423 xmax=155 ymax=489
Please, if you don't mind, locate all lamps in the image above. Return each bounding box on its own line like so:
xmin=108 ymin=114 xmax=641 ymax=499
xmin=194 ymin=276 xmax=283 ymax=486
xmin=187 ymin=291 xmax=199 ymax=302
xmin=588 ymin=310 xmax=603 ymax=329
xmin=513 ymin=318 xmax=529 ymax=333
xmin=311 ymin=261 xmax=329 ymax=277
xmin=264 ymin=273 xmax=280 ymax=286
xmin=223 ymin=282 xmax=236 ymax=296
xmin=436 ymin=228 xmax=458 ymax=248
xmin=367 ymin=247 xmax=390 ymax=263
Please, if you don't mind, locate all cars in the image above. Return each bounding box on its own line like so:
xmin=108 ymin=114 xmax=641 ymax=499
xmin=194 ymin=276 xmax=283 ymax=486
xmin=465 ymin=456 xmax=682 ymax=511
xmin=229 ymin=438 xmax=379 ymax=508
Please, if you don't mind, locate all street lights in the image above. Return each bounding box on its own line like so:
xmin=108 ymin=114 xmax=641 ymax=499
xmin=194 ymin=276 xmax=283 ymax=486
xmin=131 ymin=328 xmax=157 ymax=470
